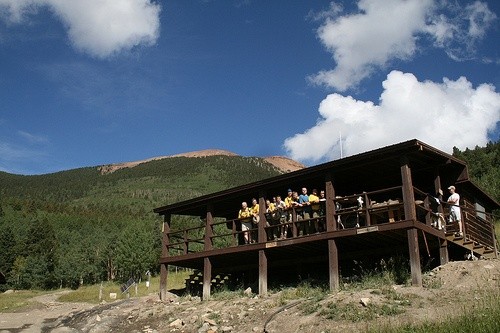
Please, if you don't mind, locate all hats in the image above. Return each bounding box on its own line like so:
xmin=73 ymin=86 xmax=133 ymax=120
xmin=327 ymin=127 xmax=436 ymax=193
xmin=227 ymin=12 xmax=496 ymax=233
xmin=447 ymin=186 xmax=455 ymax=190
xmin=288 ymin=189 xmax=292 ymax=192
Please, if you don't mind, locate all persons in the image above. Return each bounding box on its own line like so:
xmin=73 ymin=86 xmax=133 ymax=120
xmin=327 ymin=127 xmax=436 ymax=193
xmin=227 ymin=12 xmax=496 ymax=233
xmin=285 ymin=189 xmax=300 ymax=236
xmin=447 ymin=186 xmax=463 ymax=236
xmin=250 ymin=198 xmax=259 ymax=243
xmin=299 ymin=187 xmax=326 ymax=237
xmin=239 ymin=202 xmax=252 ymax=244
xmin=266 ymin=196 xmax=288 ymax=240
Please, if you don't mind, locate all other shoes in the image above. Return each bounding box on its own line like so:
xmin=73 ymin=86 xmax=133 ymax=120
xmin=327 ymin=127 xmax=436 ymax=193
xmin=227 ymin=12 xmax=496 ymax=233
xmin=455 ymin=232 xmax=463 ymax=237
